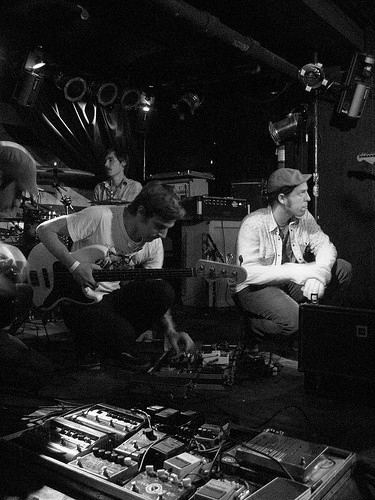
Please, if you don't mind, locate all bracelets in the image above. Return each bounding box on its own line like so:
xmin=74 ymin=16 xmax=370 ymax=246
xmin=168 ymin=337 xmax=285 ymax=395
xmin=68 ymin=260 xmax=81 ymax=273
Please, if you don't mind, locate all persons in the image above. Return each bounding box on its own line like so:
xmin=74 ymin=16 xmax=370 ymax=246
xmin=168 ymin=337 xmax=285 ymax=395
xmin=36 ymin=146 xmax=194 ymax=385
xmin=232 ymin=168 xmax=352 ymax=352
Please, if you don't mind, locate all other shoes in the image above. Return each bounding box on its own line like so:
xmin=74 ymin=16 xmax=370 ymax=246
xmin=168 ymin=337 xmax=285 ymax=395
xmin=237 ymin=318 xmax=265 ymax=354
xmin=112 ymin=349 xmax=151 ymax=371
xmin=67 ymin=350 xmax=102 ymax=371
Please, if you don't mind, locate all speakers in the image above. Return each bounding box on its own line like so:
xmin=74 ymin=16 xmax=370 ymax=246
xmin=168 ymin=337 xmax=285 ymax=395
xmin=168 ymin=220 xmax=243 ymax=308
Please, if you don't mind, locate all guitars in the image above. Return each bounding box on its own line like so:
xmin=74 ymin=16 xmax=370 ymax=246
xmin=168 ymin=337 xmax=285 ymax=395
xmin=25 ymin=241 xmax=249 ymax=312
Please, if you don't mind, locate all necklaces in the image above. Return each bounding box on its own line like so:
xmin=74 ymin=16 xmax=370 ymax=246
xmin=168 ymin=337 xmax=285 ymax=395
xmin=279 ymin=224 xmax=286 ymax=238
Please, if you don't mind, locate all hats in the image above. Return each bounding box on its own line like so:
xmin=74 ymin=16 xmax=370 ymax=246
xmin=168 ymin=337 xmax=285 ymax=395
xmin=268 ymin=168 xmax=312 ymax=194
xmin=0 ymin=140 xmax=40 ymax=196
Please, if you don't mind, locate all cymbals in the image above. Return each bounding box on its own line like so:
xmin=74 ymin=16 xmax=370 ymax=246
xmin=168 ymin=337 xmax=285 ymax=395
xmin=88 ymin=199 xmax=132 ymax=207
xmin=35 ymin=165 xmax=96 ymax=178
